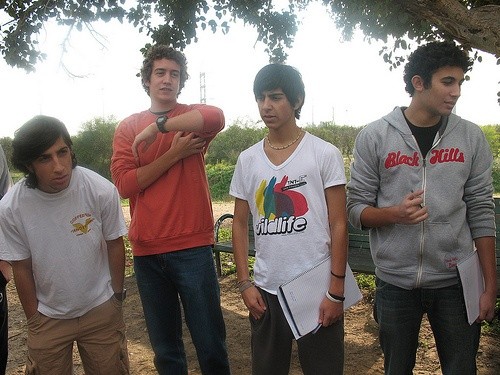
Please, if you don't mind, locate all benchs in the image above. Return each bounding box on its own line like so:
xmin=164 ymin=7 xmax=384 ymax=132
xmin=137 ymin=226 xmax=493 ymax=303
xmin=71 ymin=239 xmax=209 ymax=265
xmin=213 ymin=198 xmax=500 ymax=277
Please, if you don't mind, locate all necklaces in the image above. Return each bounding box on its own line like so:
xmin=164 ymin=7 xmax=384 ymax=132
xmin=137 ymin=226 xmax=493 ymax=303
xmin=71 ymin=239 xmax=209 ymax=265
xmin=266 ymin=127 xmax=303 ymax=150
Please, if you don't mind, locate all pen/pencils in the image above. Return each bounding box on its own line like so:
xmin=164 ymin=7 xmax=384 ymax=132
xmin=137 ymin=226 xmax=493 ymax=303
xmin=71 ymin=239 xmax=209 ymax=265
xmin=411 ymin=189 xmax=423 ymax=209
xmin=312 ymin=323 xmax=322 ymax=333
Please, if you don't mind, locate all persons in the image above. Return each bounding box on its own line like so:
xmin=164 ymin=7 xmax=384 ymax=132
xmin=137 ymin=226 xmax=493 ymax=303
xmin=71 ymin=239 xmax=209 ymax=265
xmin=229 ymin=64 xmax=349 ymax=375
xmin=110 ymin=45 xmax=230 ymax=375
xmin=1 ymin=115 xmax=130 ymax=375
xmin=345 ymin=40 xmax=496 ymax=375
xmin=0 ymin=144 xmax=15 ymax=374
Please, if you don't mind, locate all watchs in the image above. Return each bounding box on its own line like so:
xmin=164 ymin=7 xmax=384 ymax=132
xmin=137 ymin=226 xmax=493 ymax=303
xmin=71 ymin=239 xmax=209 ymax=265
xmin=156 ymin=115 xmax=169 ymax=134
xmin=113 ymin=289 xmax=126 ymax=301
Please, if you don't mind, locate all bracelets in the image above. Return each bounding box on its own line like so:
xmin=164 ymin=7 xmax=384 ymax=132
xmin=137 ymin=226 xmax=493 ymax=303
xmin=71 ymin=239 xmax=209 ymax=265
xmin=325 ymin=291 xmax=345 ymax=303
xmin=237 ymin=280 xmax=255 ymax=293
xmin=331 ymin=270 xmax=346 ymax=278
